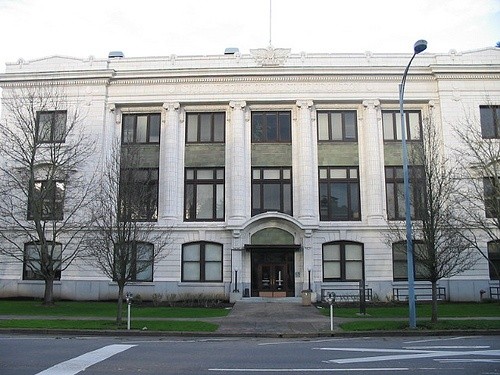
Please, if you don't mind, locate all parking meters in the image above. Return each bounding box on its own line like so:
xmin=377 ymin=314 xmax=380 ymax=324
xmin=125 ymin=291 xmax=133 ymax=330
xmin=327 ymin=291 xmax=337 ymax=333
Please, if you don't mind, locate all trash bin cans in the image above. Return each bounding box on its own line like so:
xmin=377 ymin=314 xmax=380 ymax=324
xmin=301 ymin=289 xmax=313 ymax=306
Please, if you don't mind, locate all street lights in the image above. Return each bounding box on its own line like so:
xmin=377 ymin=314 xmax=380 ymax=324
xmin=398 ymin=39 xmax=429 ymax=328
